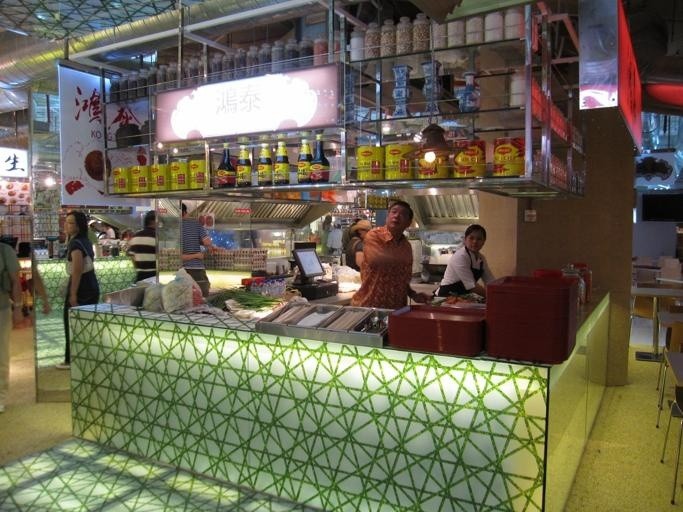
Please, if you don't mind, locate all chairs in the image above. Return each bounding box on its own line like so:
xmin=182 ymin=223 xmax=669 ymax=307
xmin=631 ymin=250 xmax=682 ymax=508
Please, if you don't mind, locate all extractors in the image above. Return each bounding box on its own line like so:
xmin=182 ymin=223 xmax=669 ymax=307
xmin=398 ymin=190 xmax=481 ymax=232
xmin=188 ymin=197 xmax=336 ymax=231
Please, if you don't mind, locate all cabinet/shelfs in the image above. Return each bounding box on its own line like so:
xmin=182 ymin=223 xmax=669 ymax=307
xmin=97 ymin=60 xmax=340 ymax=198
xmin=345 ymin=36 xmax=585 ymax=202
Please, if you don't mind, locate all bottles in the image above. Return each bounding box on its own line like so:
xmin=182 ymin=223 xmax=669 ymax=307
xmin=250 ymin=278 xmax=287 ymax=298
xmin=216 ymin=138 xmax=330 ymax=188
xmin=390 ymin=59 xmax=480 ymax=118
xmin=348 ymin=10 xmax=523 ymax=62
xmin=116 ymin=118 xmax=151 ymax=147
xmin=109 ymin=36 xmax=325 ymax=102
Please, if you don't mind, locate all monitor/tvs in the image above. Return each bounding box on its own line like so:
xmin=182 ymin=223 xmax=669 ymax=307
xmin=641 ymin=193 xmax=683 ymax=222
xmin=3 ymin=237 xmax=18 ymax=249
xmin=292 ymin=248 xmax=326 ymax=285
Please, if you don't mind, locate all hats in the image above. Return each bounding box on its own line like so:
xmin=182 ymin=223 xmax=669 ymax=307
xmin=350 ymin=220 xmax=372 ymax=233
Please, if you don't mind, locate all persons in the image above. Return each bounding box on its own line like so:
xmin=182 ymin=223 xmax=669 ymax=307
xmin=432 ymin=225 xmax=496 ymax=300
xmin=320 ymin=215 xmax=372 ymax=273
xmin=33 ymin=257 xmax=53 ymax=316
xmin=349 ymin=201 xmax=431 ymax=311
xmin=180 ymin=203 xmax=212 ymax=299
xmin=128 ymin=209 xmax=204 ymax=288
xmin=55 ymin=210 xmax=100 ymax=369
xmin=0 ymin=242 xmax=24 ymax=414
xmin=102 ymin=222 xmax=115 ymax=239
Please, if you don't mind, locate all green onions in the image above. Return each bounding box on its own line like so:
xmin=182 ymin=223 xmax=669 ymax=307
xmin=205 ymin=289 xmax=284 ymax=309
